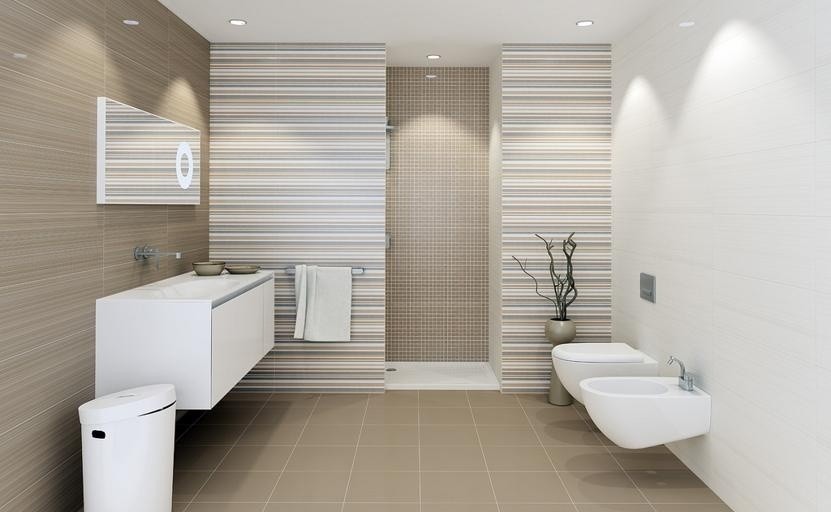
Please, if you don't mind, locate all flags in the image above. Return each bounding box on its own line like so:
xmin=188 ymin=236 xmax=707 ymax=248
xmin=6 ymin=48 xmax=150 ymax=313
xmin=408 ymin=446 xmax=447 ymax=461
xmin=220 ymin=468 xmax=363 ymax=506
xmin=544 ymin=319 xmax=577 ymax=406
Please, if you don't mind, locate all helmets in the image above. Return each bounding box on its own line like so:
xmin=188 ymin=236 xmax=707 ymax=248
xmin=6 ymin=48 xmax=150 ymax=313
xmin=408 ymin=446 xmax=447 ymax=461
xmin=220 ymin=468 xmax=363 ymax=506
xmin=224 ymin=263 xmax=260 ymax=274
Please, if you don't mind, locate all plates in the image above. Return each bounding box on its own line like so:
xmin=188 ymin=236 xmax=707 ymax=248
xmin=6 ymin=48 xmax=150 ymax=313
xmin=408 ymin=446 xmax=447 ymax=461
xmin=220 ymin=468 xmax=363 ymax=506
xmin=94 ymin=280 xmax=279 ymax=411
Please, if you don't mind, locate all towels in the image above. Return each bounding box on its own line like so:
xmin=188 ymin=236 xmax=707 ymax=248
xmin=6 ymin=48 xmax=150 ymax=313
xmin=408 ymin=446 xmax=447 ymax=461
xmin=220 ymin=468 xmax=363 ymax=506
xmin=78 ymin=383 xmax=176 ymax=511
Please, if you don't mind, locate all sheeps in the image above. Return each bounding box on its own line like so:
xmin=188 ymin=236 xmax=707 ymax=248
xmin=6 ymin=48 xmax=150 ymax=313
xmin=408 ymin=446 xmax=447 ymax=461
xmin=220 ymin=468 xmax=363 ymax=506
xmin=665 ymin=354 xmax=693 ymax=390
xmin=151 ymin=250 xmax=182 ymax=260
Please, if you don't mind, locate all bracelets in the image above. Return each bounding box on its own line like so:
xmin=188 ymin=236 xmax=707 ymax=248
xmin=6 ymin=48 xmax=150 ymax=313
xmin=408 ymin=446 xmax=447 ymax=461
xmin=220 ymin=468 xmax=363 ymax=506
xmin=192 ymin=261 xmax=224 ymax=276
